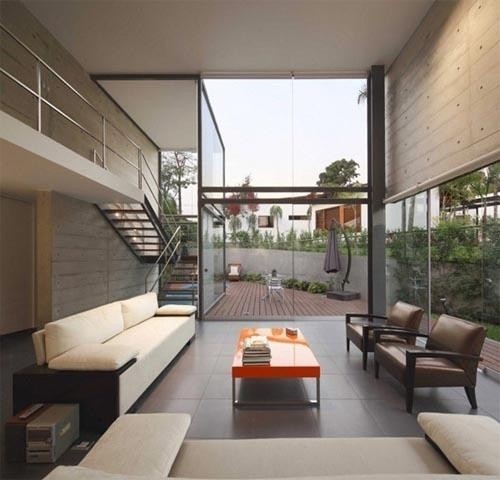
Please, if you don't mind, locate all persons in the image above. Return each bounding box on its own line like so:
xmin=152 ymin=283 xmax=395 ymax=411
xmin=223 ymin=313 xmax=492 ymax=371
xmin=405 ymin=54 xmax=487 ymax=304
xmin=262 ymin=267 xmax=284 ymax=302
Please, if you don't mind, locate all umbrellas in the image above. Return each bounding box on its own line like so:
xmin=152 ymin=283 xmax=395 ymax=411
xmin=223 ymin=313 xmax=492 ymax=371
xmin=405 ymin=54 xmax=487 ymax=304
xmin=323 ymin=217 xmax=353 ymax=292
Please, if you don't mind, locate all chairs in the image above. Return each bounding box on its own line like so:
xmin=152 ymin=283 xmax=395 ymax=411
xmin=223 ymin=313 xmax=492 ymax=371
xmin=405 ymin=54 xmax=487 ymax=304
xmin=343 ymin=299 xmax=488 ymax=415
xmin=228 ymin=263 xmax=241 ymax=282
xmin=265 ymin=273 xmax=287 ymax=304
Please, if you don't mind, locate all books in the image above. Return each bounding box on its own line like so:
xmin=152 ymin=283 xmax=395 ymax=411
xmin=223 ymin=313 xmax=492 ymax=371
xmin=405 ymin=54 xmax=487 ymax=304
xmin=242 ymin=356 xmax=272 ymax=362
xmin=242 ymin=362 xmax=270 ymax=367
xmin=243 ymin=354 xmax=270 ymax=357
xmin=251 ymin=336 xmax=268 ymax=346
xmin=244 ymin=346 xmax=271 ymax=353
xmin=244 ymin=336 xmax=270 ymax=350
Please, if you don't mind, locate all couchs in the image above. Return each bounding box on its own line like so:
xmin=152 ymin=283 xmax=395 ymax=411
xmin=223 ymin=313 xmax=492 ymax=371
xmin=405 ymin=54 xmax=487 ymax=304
xmin=14 ymin=293 xmax=198 ymax=434
xmin=40 ymin=412 xmax=500 ymax=479
